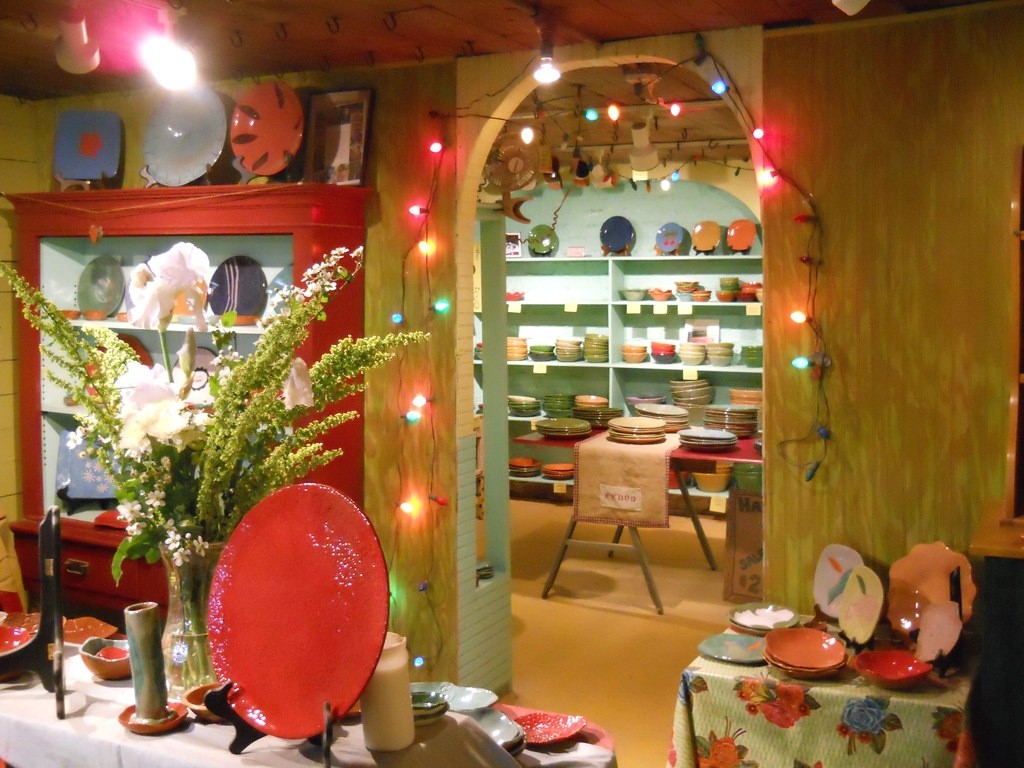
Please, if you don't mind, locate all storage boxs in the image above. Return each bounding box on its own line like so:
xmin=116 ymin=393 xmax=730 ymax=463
xmin=509 ymin=480 xmax=539 ymax=503
xmin=668 ymin=495 xmax=711 ymax=518
xmin=539 ymin=482 xmax=572 ymax=503
xmin=722 ymin=486 xmax=765 ymax=606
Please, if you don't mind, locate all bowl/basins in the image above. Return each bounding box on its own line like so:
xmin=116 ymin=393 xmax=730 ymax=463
xmin=232 ymin=314 xmax=260 ymax=326
xmin=83 ymin=310 xmax=108 ymax=320
xmin=669 ymin=460 xmax=762 ymax=492
xmin=498 ymin=379 xmax=762 ymax=411
xmin=116 ymin=312 xmax=128 ymax=322
xmin=619 ymin=277 xmax=763 ymax=302
xmin=80 ymin=635 xmax=146 ymax=682
xmin=61 ymin=309 xmax=81 ymax=320
xmin=475 ymin=334 xmax=763 ymax=369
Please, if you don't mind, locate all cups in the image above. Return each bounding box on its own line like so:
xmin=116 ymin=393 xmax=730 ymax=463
xmin=360 ymin=627 xmax=415 ymax=750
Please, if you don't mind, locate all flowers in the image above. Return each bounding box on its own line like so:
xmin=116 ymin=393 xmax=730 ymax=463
xmin=0 ymin=244 xmax=433 ymax=689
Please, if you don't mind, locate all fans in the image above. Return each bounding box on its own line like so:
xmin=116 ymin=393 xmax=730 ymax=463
xmin=480 ymin=130 xmax=542 ymax=223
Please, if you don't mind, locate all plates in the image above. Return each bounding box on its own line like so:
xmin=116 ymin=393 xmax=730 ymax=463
xmin=634 ymin=403 xmax=687 ymax=415
xmin=536 ymin=412 xmax=758 ymax=449
xmin=54 ymin=79 xmax=304 ymax=186
xmin=346 ymin=681 xmax=587 ymax=757
xmin=0 ymin=611 xmax=118 ymax=644
xmin=207 ymin=484 xmax=390 ymax=741
xmin=506 ymin=291 xmax=525 ymax=300
xmin=527 ymin=216 xmax=756 ymax=253
xmin=509 ymin=457 xmax=574 ymax=479
xmin=697 ymin=541 xmax=976 ymax=689
xmin=78 ymin=249 xmax=267 ymax=411
xmin=57 ymin=430 xmax=131 ymax=498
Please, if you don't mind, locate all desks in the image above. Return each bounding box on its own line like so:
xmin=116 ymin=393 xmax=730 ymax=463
xmin=512 ymin=424 xmax=763 ymax=616
xmin=0 ymin=642 xmax=616 ymax=768
xmin=664 ymin=611 xmax=974 ymax=768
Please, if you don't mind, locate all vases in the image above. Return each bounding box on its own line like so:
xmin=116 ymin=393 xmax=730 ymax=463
xmin=159 ymin=541 xmax=224 ymax=699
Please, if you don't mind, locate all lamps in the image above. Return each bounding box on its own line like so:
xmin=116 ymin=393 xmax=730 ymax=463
xmin=51 ymin=0 xmax=101 ymax=76
xmin=627 ymin=109 xmax=659 ymax=171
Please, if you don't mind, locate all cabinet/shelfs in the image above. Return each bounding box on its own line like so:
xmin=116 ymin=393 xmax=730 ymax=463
xmin=0 ymin=183 xmax=377 ymax=641
xmin=473 ymin=201 xmax=527 ymax=595
xmin=965 ymin=140 xmax=1024 ymax=768
xmin=504 ymin=254 xmax=766 ymax=517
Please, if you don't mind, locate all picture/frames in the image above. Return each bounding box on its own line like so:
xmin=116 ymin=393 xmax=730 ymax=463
xmin=505 ymin=232 xmax=522 ymax=258
xmin=296 ymin=86 xmax=375 ymax=187
